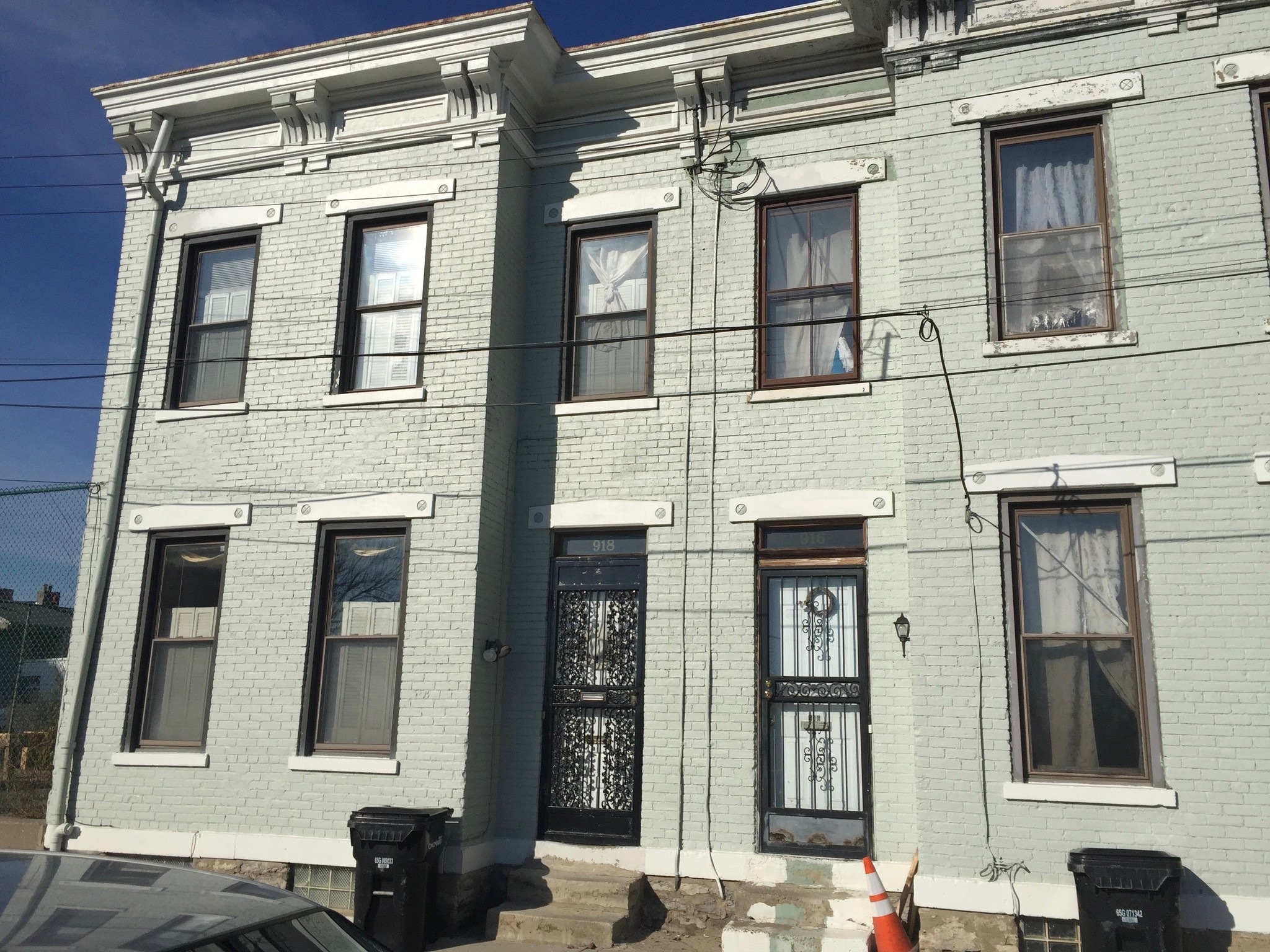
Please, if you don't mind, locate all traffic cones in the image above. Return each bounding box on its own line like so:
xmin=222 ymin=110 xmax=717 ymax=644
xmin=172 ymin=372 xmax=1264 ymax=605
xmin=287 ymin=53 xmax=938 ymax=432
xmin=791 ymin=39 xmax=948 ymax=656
xmin=863 ymin=856 xmax=913 ymax=952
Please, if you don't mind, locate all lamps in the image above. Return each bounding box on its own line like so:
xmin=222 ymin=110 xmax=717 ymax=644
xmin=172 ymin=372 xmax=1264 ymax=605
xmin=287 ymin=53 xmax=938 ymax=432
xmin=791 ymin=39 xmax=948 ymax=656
xmin=483 ymin=638 xmax=512 ymax=663
xmin=894 ymin=613 xmax=911 ymax=658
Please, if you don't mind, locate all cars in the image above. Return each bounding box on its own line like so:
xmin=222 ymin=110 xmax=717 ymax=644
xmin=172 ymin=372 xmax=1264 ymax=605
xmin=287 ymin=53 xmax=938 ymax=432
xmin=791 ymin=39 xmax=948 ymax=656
xmin=0 ymin=849 xmax=398 ymax=952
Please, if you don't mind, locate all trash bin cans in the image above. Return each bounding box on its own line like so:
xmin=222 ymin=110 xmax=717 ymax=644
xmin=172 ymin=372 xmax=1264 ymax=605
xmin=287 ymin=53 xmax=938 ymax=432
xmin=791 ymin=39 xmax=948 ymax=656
xmin=1068 ymin=846 xmax=1179 ymax=952
xmin=347 ymin=806 xmax=454 ymax=952
